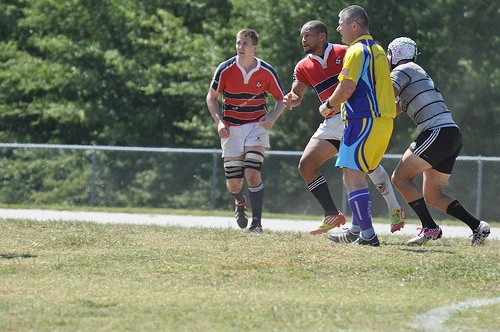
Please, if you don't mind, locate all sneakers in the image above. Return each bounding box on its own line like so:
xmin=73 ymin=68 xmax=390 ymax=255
xmin=235 ymin=195 xmax=247 ymax=228
xmin=309 ymin=211 xmax=346 ymax=235
xmin=390 ymin=207 xmax=405 ymax=232
xmin=352 ymin=232 xmax=379 ymax=246
xmin=250 ymin=221 xmax=263 ymax=233
xmin=469 ymin=221 xmax=492 ymax=246
xmin=328 ymin=229 xmax=359 ymax=244
xmin=407 ymin=225 xmax=442 ymax=246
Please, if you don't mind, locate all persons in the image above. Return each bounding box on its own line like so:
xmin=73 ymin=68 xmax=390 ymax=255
xmin=387 ymin=36 xmax=491 ymax=247
xmin=206 ymin=29 xmax=286 ymax=234
xmin=319 ymin=4 xmax=397 ymax=248
xmin=282 ymin=20 xmax=407 ymax=235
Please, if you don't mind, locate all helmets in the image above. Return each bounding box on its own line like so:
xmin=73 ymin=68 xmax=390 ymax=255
xmin=388 ymin=36 xmax=416 ymax=66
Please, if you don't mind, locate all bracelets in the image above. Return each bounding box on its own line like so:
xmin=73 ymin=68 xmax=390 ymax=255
xmin=325 ymin=98 xmax=333 ymax=109
